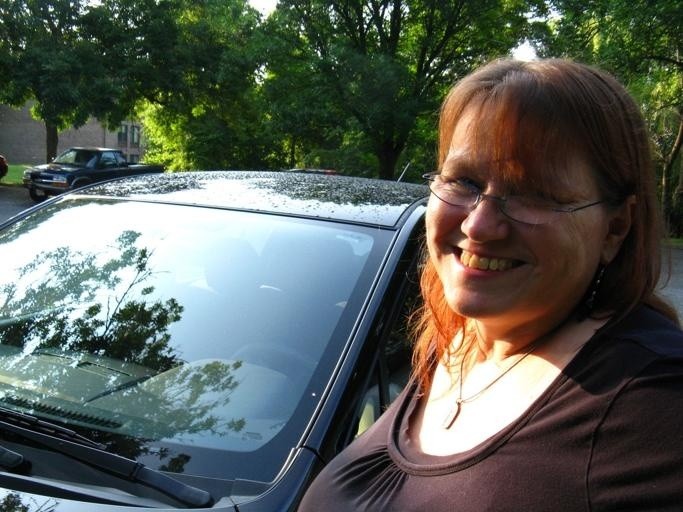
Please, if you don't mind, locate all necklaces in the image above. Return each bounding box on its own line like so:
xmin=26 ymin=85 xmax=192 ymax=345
xmin=444 ymin=326 xmax=562 ymax=429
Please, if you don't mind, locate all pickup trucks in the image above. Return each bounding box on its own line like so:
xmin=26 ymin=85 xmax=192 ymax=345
xmin=20 ymin=143 xmax=166 ymax=206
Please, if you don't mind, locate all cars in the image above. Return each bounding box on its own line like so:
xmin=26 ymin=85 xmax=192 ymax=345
xmin=285 ymin=167 xmax=337 ymax=174
xmin=1 ymin=165 xmax=441 ymax=511
xmin=0 ymin=153 xmax=9 ymax=181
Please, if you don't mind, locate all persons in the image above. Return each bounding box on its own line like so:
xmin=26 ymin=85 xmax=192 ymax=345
xmin=296 ymin=55 xmax=683 ymax=512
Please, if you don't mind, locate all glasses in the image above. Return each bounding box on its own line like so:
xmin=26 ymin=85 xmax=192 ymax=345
xmin=421 ymin=170 xmax=608 ymax=227
